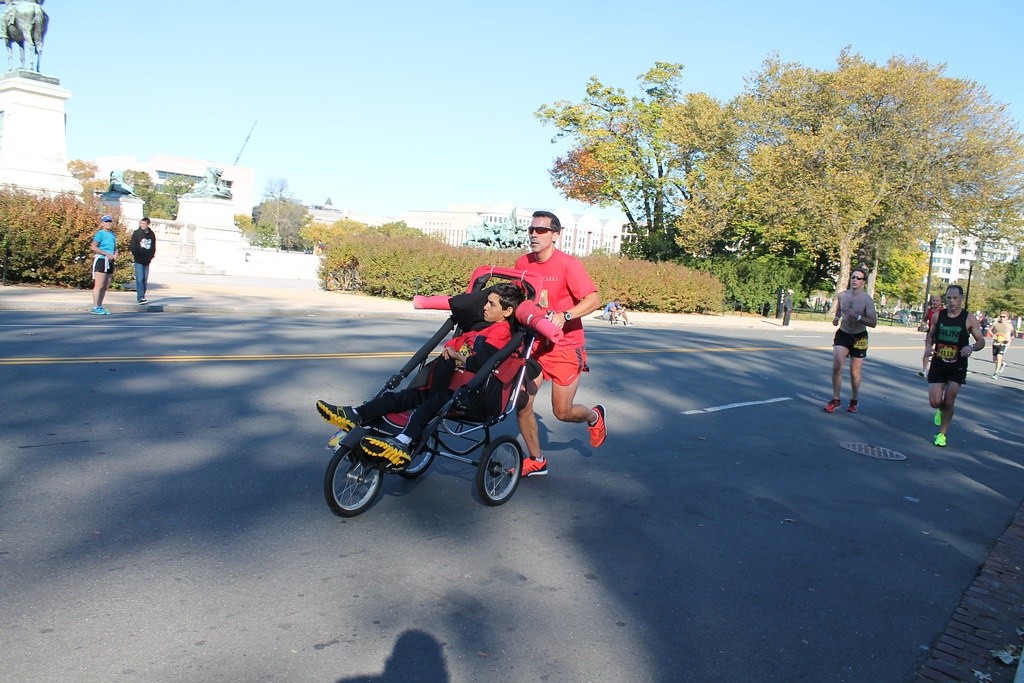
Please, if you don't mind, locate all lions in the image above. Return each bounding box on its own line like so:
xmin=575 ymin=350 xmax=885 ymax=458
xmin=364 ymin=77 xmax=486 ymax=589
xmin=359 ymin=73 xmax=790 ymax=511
xmin=194 ymin=166 xmax=231 ymax=193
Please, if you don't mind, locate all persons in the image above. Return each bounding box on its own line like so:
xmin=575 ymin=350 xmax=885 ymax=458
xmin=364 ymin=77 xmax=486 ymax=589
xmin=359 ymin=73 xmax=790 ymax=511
xmin=129 ymin=218 xmax=156 ymax=305
xmin=0 ymin=0 xmax=46 ymax=54
xmin=89 ymin=215 xmax=118 ymax=315
xmin=989 ymin=311 xmax=1016 ymax=380
xmin=782 ymin=289 xmax=794 ymax=327
xmin=317 ymin=282 xmax=525 ymax=466
xmin=508 ymin=211 xmax=606 ymax=478
xmin=603 ymin=298 xmax=635 ymax=326
xmin=981 ymin=314 xmax=1016 ymax=341
xmin=927 ymin=285 xmax=985 ymax=446
xmin=918 ymin=296 xmax=946 ymax=378
xmin=824 ymin=269 xmax=877 ymax=411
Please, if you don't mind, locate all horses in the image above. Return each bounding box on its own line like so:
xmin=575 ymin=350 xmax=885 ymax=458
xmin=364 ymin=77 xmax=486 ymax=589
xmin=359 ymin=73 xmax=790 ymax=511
xmin=3 ymin=2 xmax=49 ymax=73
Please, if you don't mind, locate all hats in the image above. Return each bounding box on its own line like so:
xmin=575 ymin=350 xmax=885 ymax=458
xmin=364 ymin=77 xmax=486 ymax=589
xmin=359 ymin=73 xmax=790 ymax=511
xmin=100 ymin=216 xmax=112 ymax=222
xmin=788 ymin=289 xmax=794 ymax=294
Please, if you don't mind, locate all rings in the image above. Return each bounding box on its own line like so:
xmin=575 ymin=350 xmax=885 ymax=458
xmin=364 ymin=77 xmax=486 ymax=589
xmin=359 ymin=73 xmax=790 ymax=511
xmin=968 ymin=353 xmax=969 ymax=355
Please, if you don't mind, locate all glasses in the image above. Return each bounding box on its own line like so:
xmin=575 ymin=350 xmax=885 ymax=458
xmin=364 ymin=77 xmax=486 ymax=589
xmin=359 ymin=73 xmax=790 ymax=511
xmin=851 ymin=275 xmax=864 ymax=281
xmin=1000 ymin=316 xmax=1007 ymax=319
xmin=528 ymin=226 xmax=556 ymax=235
xmin=946 ymin=295 xmax=962 ymax=302
xmin=104 ymin=220 xmax=112 ymax=223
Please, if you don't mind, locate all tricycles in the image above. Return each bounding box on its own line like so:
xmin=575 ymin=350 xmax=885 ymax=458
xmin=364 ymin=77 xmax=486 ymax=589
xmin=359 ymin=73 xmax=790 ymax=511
xmin=324 ymin=266 xmax=554 ymax=519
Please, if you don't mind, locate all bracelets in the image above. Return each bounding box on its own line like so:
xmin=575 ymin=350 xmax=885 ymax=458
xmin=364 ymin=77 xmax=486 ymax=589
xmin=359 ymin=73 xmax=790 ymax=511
xmin=857 ymin=314 xmax=862 ymax=322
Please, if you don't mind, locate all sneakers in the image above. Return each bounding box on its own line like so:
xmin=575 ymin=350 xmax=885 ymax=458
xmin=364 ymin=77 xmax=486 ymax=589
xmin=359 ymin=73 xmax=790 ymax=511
xmin=934 ymin=432 xmax=946 ymax=446
xmin=991 ymin=373 xmax=998 ymax=380
xmin=824 ymin=399 xmax=842 ymax=412
xmin=999 ymin=362 xmax=1006 ymax=373
xmin=361 ymin=435 xmax=412 ymax=468
xmin=586 ymin=405 xmax=607 ymax=448
xmin=317 ymin=400 xmax=362 ymax=432
xmin=934 ymin=407 xmax=942 ymax=426
xmin=846 ymin=398 xmax=859 ymax=412
xmin=508 ymin=456 xmax=548 ymax=477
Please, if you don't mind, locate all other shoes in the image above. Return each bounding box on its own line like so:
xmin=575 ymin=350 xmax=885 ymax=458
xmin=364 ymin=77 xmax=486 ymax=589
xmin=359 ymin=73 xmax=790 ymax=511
xmin=138 ymin=299 xmax=149 ymax=305
xmin=99 ymin=307 xmax=112 ymax=315
xmin=611 ymin=321 xmax=619 ymax=326
xmin=90 ymin=307 xmax=106 ymax=315
xmin=626 ymin=322 xmax=635 ymax=326
xmin=919 ymin=369 xmax=928 ymax=378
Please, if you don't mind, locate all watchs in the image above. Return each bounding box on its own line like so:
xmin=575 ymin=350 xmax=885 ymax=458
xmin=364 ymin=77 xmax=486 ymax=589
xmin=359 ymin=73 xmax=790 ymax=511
xmin=971 ymin=344 xmax=975 ymax=350
xmin=563 ymin=310 xmax=571 ymax=322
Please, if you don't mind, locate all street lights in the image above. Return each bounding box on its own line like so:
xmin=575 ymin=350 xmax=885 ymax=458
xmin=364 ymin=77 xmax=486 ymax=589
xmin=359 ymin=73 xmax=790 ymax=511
xmin=918 ymin=229 xmax=940 ymax=332
xmin=965 ymin=255 xmax=975 ymax=310
